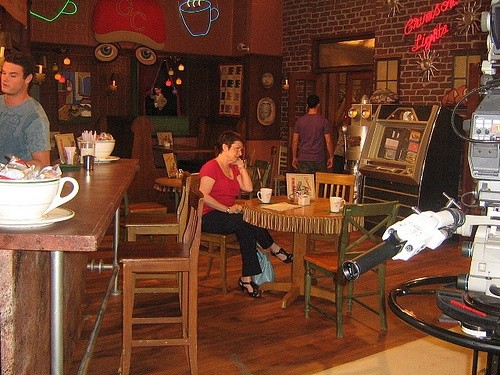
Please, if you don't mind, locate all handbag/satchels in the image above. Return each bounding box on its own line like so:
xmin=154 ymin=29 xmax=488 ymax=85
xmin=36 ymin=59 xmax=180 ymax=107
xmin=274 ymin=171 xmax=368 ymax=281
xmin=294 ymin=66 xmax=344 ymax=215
xmin=254 ymin=249 xmax=276 ymax=285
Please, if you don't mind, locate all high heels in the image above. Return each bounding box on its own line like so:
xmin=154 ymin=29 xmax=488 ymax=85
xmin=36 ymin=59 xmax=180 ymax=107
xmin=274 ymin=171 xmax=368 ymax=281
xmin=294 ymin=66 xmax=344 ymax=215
xmin=270 ymin=248 xmax=294 ymax=263
xmin=238 ymin=278 xmax=261 ymax=298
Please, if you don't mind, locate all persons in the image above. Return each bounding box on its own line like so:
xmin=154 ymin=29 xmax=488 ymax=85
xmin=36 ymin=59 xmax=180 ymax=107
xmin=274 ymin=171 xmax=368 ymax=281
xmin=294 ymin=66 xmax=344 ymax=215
xmin=0 ymin=50 xmax=52 ymax=171
xmin=291 ymin=93 xmax=335 ymax=174
xmin=195 ymin=129 xmax=294 ymax=299
xmin=148 ymin=84 xmax=172 ymax=116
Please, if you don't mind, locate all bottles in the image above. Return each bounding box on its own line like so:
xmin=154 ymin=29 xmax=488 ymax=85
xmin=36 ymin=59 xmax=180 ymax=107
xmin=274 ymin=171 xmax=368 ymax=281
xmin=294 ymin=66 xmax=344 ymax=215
xmin=178 ymin=169 xmax=183 ymax=178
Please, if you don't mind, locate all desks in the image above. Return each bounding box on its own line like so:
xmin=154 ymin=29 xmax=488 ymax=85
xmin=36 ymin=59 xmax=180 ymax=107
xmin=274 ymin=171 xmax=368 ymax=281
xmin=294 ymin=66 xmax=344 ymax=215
xmin=152 ymin=146 xmax=215 ymax=167
xmin=0 ymin=158 xmax=143 ymax=375
xmin=153 ymin=176 xmax=182 ymax=213
xmin=243 ymin=196 xmax=364 ymax=310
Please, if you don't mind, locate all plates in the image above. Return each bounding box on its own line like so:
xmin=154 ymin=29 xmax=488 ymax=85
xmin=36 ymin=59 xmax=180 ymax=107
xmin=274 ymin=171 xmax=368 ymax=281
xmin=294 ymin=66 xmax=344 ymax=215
xmin=78 ymin=155 xmax=120 ymax=164
xmin=0 ymin=206 xmax=74 ymax=231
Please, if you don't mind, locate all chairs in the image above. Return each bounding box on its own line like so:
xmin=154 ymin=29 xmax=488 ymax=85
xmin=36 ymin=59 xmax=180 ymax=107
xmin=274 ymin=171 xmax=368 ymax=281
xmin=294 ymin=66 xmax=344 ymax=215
xmin=118 ymin=145 xmax=396 ymax=375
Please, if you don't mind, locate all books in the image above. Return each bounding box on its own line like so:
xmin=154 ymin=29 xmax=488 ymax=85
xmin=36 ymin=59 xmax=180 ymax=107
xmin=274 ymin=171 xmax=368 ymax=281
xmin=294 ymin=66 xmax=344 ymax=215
xmin=261 ymin=202 xmax=303 ymax=213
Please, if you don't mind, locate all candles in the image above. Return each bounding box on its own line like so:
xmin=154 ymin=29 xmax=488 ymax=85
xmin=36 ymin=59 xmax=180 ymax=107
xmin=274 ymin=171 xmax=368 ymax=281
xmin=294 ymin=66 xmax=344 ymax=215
xmin=113 ymin=80 xmax=115 ymax=86
xmin=286 ymin=80 xmax=288 ymax=85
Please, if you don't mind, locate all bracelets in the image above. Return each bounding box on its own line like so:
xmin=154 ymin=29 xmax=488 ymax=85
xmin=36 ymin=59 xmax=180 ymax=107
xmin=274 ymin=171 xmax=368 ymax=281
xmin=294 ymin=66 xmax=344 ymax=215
xmin=225 ymin=206 xmax=232 ymax=213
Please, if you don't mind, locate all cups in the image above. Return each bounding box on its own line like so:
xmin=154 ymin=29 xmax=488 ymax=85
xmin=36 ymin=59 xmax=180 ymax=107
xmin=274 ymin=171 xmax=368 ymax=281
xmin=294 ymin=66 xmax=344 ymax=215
xmin=257 ymin=187 xmax=273 ymax=203
xmin=76 ymin=138 xmax=115 ymax=159
xmin=329 ymin=197 xmax=347 ymax=213
xmin=0 ymin=176 xmax=81 ymax=221
xmin=83 ymin=156 xmax=94 ymax=169
